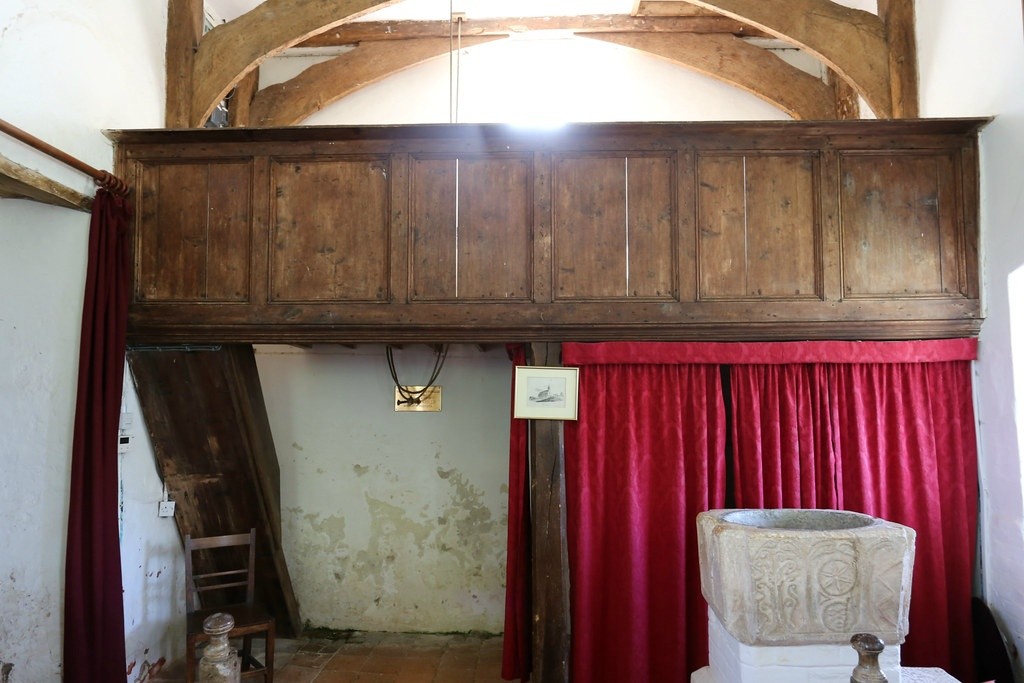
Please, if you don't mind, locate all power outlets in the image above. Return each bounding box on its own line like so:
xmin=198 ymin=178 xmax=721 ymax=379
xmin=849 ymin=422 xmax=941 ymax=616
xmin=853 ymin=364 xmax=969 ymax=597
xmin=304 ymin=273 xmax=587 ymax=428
xmin=159 ymin=502 xmax=175 ymax=516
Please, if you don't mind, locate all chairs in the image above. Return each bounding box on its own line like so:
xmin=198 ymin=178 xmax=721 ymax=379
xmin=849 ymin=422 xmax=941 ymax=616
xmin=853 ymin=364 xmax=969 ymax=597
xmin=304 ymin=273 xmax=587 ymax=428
xmin=185 ymin=528 xmax=275 ymax=683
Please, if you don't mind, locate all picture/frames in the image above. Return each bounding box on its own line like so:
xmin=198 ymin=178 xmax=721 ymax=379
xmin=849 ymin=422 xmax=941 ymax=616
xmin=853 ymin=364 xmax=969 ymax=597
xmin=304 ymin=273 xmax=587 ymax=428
xmin=514 ymin=365 xmax=579 ymax=420
